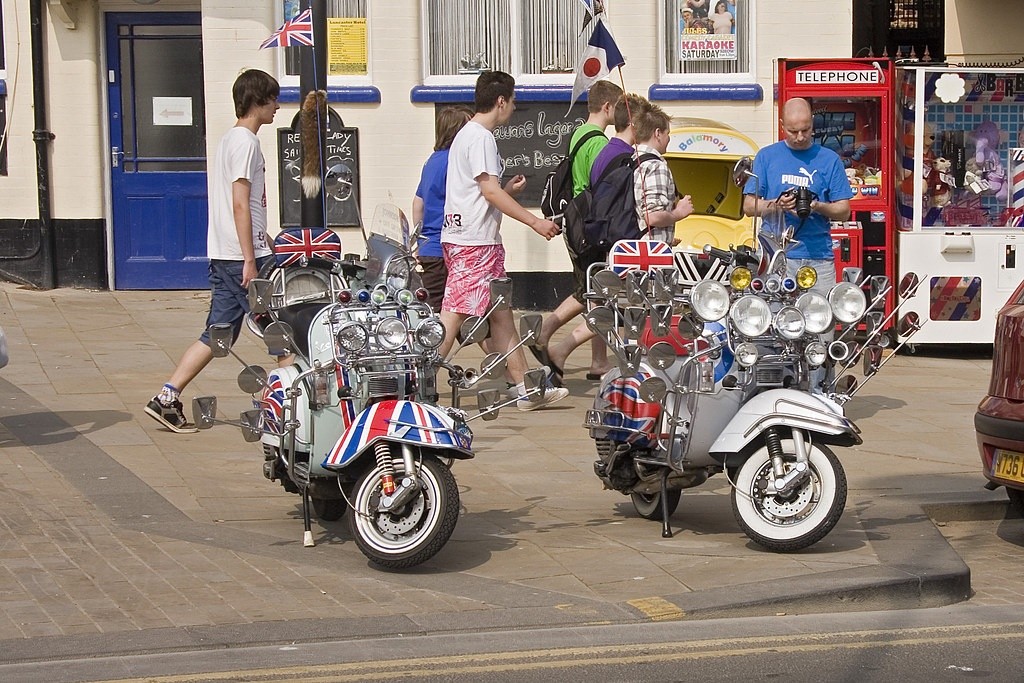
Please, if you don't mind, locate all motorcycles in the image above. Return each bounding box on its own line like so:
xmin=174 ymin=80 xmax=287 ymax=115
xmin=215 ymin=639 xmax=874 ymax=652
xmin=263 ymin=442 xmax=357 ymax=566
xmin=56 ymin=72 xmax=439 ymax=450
xmin=579 ymin=155 xmax=933 ymax=554
xmin=191 ymin=161 xmax=550 ymax=569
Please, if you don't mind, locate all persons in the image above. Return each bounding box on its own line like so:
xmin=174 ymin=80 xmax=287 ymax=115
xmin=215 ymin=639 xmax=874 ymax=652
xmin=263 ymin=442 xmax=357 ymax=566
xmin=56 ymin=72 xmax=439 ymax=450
xmin=528 ymin=79 xmax=652 ymax=388
xmin=145 ymin=69 xmax=296 ymax=433
xmin=743 ymin=98 xmax=854 ymax=393
xmin=412 ymin=107 xmax=553 ymax=399
xmin=682 ymin=0 xmax=735 ymax=34
xmin=628 ymin=110 xmax=694 ymax=248
xmin=436 ymin=72 xmax=569 ymax=411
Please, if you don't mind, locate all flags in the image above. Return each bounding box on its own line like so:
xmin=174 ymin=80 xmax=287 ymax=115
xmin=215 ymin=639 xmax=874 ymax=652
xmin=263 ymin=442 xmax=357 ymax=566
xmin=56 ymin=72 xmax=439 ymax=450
xmin=259 ymin=9 xmax=313 ymax=49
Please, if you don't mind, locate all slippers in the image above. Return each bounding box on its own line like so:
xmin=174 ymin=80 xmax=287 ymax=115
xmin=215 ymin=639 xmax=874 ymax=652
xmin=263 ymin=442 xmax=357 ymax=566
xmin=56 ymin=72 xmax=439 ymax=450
xmin=586 ymin=365 xmax=617 ymax=379
xmin=530 ymin=344 xmax=542 ymax=359
xmin=543 ymin=346 xmax=563 ymax=385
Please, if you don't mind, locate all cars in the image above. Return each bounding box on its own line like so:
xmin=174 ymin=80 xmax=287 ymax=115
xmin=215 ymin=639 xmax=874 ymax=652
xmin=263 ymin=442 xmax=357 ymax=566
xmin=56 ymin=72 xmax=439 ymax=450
xmin=973 ymin=280 xmax=1024 ymax=509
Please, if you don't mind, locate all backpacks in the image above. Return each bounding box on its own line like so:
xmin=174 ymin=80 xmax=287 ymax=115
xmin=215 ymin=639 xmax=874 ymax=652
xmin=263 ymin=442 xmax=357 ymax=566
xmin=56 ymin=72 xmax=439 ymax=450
xmin=541 ymin=129 xmax=609 ymax=235
xmin=561 ymin=152 xmax=633 ymax=270
xmin=581 ymin=153 xmax=663 ymax=251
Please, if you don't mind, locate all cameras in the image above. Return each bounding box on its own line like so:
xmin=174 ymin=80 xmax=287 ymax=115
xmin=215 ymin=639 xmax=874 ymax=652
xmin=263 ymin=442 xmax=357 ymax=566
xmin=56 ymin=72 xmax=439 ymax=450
xmin=788 ymin=186 xmax=813 ymax=218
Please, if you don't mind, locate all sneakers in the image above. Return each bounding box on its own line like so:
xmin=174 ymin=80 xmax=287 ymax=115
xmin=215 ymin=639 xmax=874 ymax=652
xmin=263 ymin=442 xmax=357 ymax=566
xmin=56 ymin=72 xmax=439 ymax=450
xmin=505 ymin=366 xmax=554 ymax=400
xmin=144 ymin=396 xmax=198 ymax=433
xmin=517 ymin=387 xmax=569 ymax=411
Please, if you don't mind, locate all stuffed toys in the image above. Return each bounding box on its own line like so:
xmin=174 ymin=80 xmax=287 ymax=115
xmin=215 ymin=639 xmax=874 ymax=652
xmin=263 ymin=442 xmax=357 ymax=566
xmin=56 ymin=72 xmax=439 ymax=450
xmin=896 ymin=121 xmax=1024 ymax=227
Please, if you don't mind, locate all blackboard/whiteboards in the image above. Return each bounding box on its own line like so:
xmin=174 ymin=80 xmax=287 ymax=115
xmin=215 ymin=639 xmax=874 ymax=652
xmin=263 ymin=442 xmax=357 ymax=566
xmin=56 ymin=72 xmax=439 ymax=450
xmin=435 ymin=102 xmax=589 ymax=208
xmin=276 ymin=106 xmax=361 ymax=227
